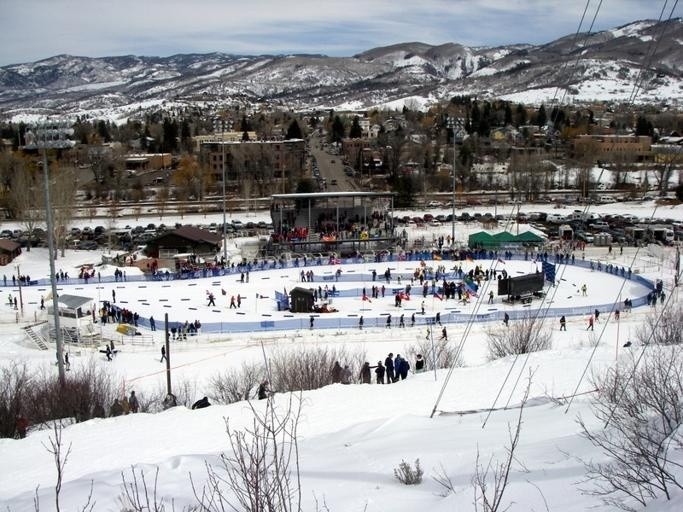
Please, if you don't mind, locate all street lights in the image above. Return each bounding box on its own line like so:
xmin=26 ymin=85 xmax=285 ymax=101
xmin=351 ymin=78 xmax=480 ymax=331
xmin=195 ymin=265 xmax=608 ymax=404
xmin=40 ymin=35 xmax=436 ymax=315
xmin=12 ymin=262 xmax=24 ymax=318
xmin=445 ymin=115 xmax=473 ymax=250
xmin=20 ymin=112 xmax=78 ymax=380
xmin=210 ymin=117 xmax=237 ymax=260
xmin=145 ymin=135 xmax=165 ymax=167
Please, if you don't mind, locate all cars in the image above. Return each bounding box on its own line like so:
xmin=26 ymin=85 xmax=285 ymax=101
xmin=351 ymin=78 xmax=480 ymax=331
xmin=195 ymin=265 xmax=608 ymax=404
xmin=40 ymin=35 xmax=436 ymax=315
xmin=330 ymin=160 xmax=336 ymax=163
xmin=306 ymin=148 xmax=326 ymax=191
xmin=313 ymin=139 xmax=338 ymax=155
xmin=329 ymin=179 xmax=338 ymax=186
xmin=172 ymin=253 xmax=205 ymax=264
xmin=256 ymin=251 xmax=340 ymax=262
xmin=0 ymin=221 xmax=274 ymax=249
xmin=412 ymin=194 xmax=677 ymax=207
xmin=385 ymin=210 xmax=683 ymax=248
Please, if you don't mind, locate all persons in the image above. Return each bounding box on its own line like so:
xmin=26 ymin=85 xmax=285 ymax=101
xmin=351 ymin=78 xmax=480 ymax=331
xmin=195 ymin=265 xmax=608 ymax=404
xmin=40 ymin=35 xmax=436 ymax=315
xmin=259 ymin=381 xmax=269 ymax=400
xmin=92 ymin=391 xmax=140 ymax=418
xmin=192 ymin=397 xmax=211 ymax=410
xmin=16 ymin=414 xmax=29 ymax=439
xmin=0 ymin=210 xmax=665 ymax=371
xmin=330 ymin=353 xmax=424 ymax=386
